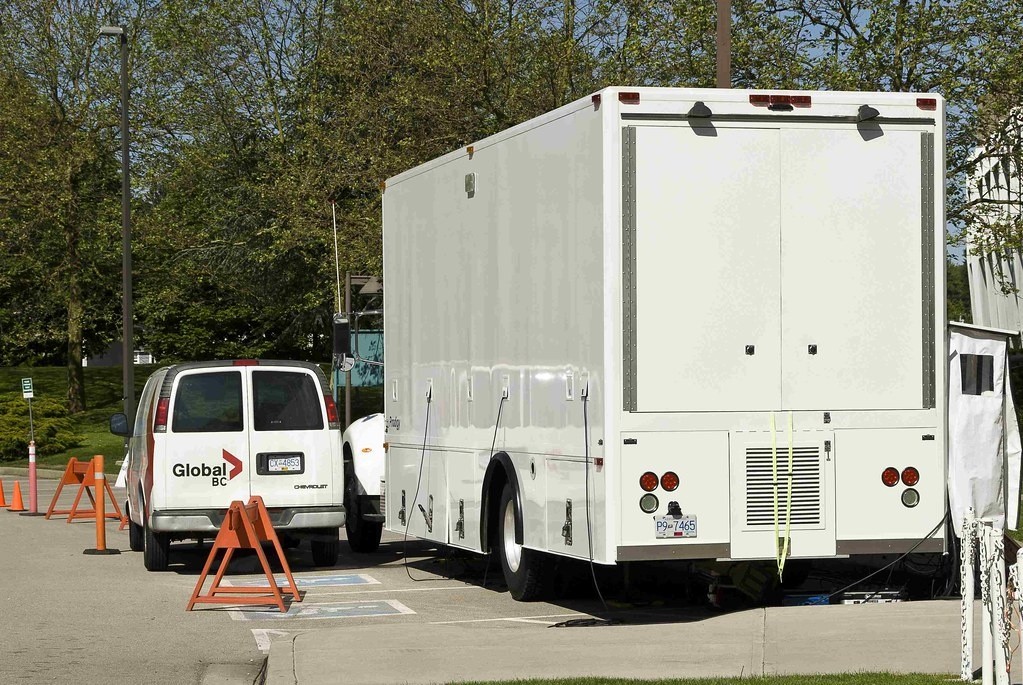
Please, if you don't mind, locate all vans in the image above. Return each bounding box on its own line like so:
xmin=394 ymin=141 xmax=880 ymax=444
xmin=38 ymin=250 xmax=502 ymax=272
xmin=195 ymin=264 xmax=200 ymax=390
xmin=108 ymin=356 xmax=347 ymax=573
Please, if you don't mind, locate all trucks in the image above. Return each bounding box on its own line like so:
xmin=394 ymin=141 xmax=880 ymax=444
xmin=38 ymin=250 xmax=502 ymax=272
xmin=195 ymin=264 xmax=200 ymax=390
xmin=342 ymin=79 xmax=948 ymax=616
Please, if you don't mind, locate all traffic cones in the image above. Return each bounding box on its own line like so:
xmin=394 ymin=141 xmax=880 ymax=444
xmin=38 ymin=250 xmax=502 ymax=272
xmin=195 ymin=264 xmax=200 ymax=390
xmin=8 ymin=481 xmax=28 ymax=513
xmin=0 ymin=478 xmax=9 ymax=508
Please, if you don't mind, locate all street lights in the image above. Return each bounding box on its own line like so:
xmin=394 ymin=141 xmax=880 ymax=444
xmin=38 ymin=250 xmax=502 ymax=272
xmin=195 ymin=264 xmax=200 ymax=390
xmin=345 ymin=268 xmax=386 ymax=428
xmin=101 ymin=24 xmax=137 ymax=444
